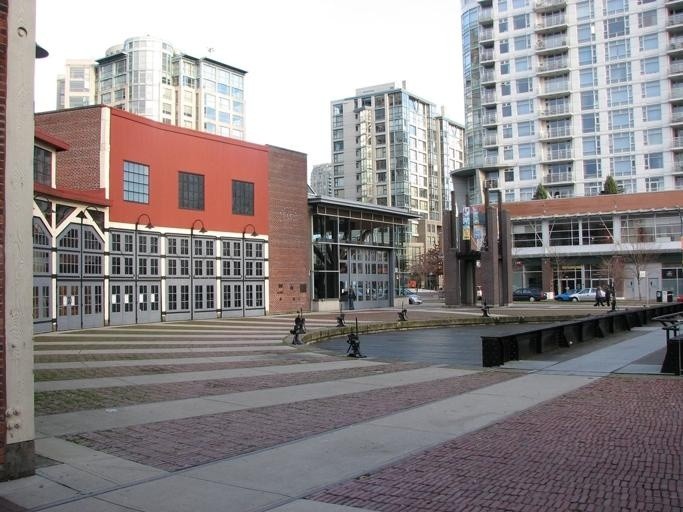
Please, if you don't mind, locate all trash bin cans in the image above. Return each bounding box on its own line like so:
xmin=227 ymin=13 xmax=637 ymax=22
xmin=656 ymin=291 xmax=672 ymax=302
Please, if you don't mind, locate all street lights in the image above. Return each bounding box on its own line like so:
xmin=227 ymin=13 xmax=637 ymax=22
xmin=188 ymin=218 xmax=208 ymax=320
xmin=132 ymin=212 xmax=155 ymax=324
xmin=240 ymin=223 xmax=257 ymax=317
xmin=77 ymin=203 xmax=103 ymax=328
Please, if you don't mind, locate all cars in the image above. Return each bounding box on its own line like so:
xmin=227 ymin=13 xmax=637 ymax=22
xmin=394 ymin=287 xmax=423 ymax=306
xmin=512 ymin=287 xmax=548 ymax=303
xmin=555 ymin=288 xmax=613 ymax=302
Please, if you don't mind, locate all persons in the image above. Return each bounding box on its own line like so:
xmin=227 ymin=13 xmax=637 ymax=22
xmin=593 ymin=286 xmax=605 ymax=307
xmin=349 ymin=285 xmax=355 ymax=310
xmin=605 ymin=286 xmax=611 ymax=307
